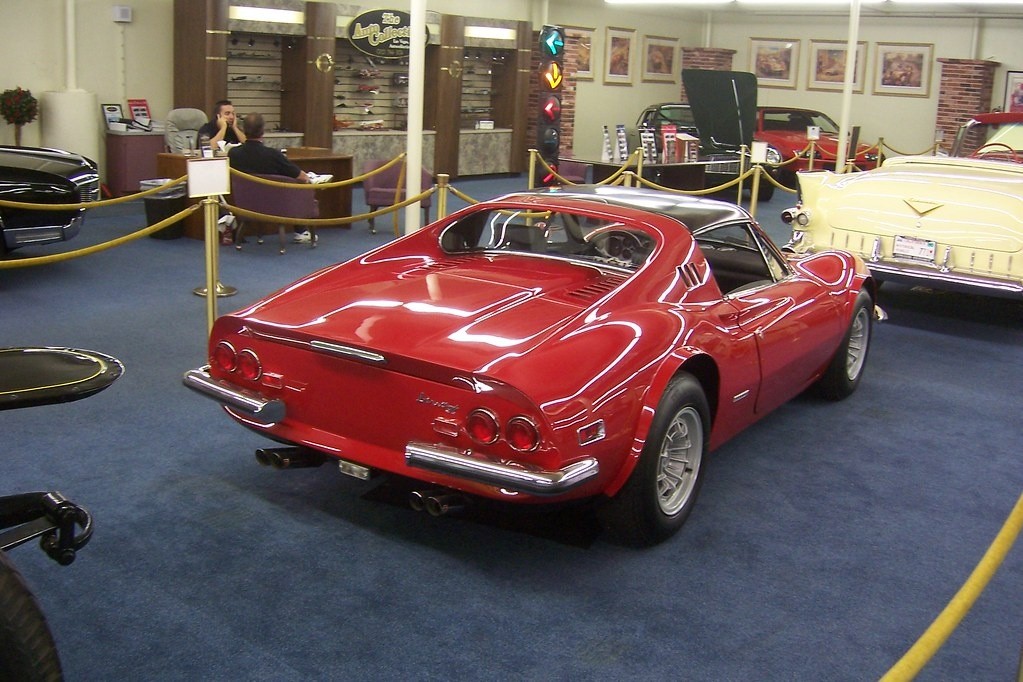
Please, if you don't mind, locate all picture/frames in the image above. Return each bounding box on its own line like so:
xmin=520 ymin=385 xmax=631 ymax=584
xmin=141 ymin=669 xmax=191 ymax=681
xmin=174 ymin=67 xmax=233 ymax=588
xmin=603 ymin=24 xmax=637 ymax=85
xmin=559 ymin=24 xmax=598 ymax=80
xmin=807 ymin=38 xmax=869 ymax=93
xmin=747 ymin=36 xmax=801 ymax=89
xmin=872 ymin=41 xmax=934 ymax=99
xmin=639 ymin=35 xmax=681 ymax=84
xmin=1003 ymin=70 xmax=1023 ymax=112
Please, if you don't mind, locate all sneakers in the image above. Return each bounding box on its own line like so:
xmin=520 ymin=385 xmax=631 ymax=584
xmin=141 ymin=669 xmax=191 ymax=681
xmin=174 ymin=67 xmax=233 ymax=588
xmin=294 ymin=230 xmax=318 ymax=242
xmin=307 ymin=172 xmax=334 ymax=184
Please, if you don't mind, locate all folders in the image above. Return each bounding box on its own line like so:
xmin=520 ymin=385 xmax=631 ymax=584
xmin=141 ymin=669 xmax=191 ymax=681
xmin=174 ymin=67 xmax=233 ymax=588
xmin=109 ymin=118 xmax=151 ymax=133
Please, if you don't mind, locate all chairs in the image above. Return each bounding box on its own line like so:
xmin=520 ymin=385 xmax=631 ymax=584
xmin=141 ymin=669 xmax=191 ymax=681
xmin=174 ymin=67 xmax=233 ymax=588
xmin=786 ymin=115 xmax=817 ymax=131
xmin=495 ymin=223 xmax=552 ymax=253
xmin=164 ymin=109 xmax=208 ymax=151
xmin=233 ymin=170 xmax=321 ymax=256
xmin=361 ymin=158 xmax=438 ymax=232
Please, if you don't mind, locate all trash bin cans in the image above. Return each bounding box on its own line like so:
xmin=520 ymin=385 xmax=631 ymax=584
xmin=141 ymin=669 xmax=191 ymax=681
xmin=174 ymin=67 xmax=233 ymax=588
xmin=140 ymin=178 xmax=187 ymax=239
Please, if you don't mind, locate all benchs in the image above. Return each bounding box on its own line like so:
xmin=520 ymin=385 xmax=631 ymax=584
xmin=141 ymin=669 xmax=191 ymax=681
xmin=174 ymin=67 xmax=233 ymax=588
xmin=762 ymin=119 xmax=786 ymax=131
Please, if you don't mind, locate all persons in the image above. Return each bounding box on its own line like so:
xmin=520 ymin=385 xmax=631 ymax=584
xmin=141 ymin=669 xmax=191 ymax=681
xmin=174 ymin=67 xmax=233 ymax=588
xmin=228 ymin=111 xmax=310 ymax=182
xmin=196 ymin=100 xmax=246 ymax=150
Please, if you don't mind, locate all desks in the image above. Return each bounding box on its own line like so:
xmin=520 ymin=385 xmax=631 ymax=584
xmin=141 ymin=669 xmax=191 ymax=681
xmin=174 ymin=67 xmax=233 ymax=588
xmin=561 ymin=154 xmax=742 ymax=196
xmin=155 ymin=146 xmax=353 ymax=236
xmin=107 ymin=129 xmax=203 ymax=193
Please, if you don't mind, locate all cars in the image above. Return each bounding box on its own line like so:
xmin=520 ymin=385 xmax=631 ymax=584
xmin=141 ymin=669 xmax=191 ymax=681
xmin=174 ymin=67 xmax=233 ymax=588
xmin=1 ymin=143 xmax=99 ymax=260
xmin=756 ymin=105 xmax=885 ymax=180
xmin=781 ymin=112 xmax=1023 ymax=302
xmin=634 ymin=68 xmax=782 ymax=202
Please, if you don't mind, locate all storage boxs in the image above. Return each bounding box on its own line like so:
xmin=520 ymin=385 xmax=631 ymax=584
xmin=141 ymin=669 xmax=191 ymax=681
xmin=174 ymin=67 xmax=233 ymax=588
xmin=676 ymin=130 xmax=700 ymax=159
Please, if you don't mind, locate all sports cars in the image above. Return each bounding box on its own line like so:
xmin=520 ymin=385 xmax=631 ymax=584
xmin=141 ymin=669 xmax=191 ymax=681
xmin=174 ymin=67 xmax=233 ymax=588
xmin=179 ymin=183 xmax=877 ymax=540
